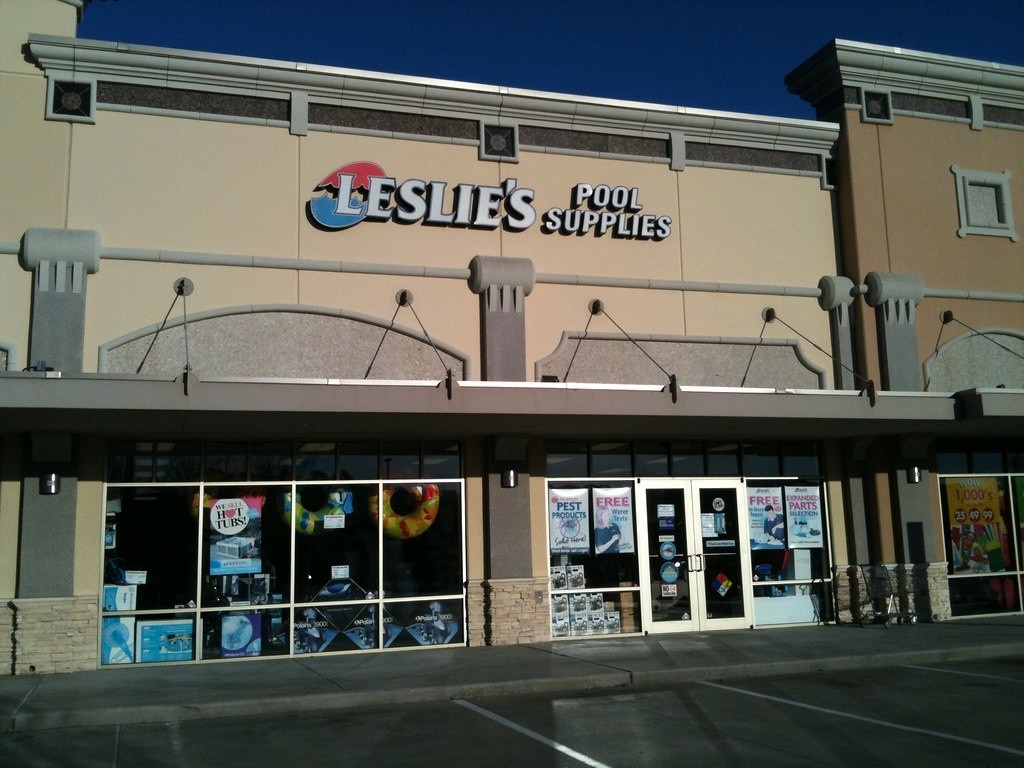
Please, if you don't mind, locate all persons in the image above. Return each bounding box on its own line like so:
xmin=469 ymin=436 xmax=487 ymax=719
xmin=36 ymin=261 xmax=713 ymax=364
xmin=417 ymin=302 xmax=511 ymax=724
xmin=764 ymin=505 xmax=785 ymax=542
xmin=595 ymin=505 xmax=621 ymax=554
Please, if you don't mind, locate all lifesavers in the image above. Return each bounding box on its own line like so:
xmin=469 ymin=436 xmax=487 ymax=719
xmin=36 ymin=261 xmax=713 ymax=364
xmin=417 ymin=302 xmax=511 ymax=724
xmin=369 ymin=484 xmax=440 ymax=538
xmin=276 ymin=487 xmax=352 ymax=532
